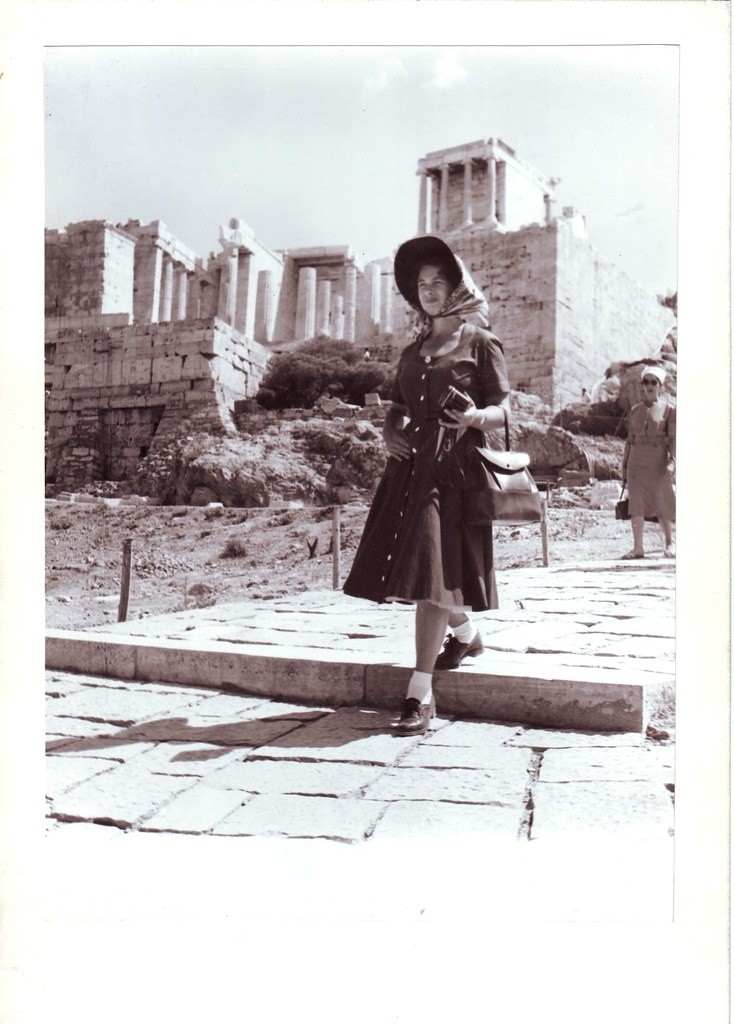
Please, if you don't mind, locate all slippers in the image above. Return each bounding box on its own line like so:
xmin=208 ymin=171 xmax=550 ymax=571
xmin=664 ymin=549 xmax=675 ymax=559
xmin=621 ymin=551 xmax=644 ymax=560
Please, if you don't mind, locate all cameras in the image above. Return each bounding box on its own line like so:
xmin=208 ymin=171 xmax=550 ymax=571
xmin=439 ymin=386 xmax=470 ymax=423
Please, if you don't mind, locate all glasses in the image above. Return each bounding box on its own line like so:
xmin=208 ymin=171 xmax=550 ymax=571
xmin=643 ymin=379 xmax=658 ymax=386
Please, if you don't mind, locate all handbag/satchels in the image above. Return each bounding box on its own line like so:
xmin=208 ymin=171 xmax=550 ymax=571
xmin=461 ymin=404 xmax=542 ymax=526
xmin=616 ymin=480 xmax=631 ymax=521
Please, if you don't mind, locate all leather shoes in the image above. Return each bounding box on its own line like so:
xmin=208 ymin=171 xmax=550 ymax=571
xmin=434 ymin=629 xmax=486 ymax=670
xmin=395 ymin=693 xmax=436 ymax=736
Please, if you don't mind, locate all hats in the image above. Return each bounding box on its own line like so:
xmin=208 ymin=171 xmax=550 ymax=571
xmin=394 ymin=236 xmax=487 ymax=320
xmin=641 ymin=367 xmax=667 ymax=386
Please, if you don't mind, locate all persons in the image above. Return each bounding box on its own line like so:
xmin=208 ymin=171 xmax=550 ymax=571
xmin=597 ymin=367 xmax=621 ymax=402
xmin=343 ymin=236 xmax=512 ymax=737
xmin=579 ymin=388 xmax=594 ymax=403
xmin=620 ymin=365 xmax=676 ymax=560
xmin=362 ymin=347 xmax=371 ymax=362
